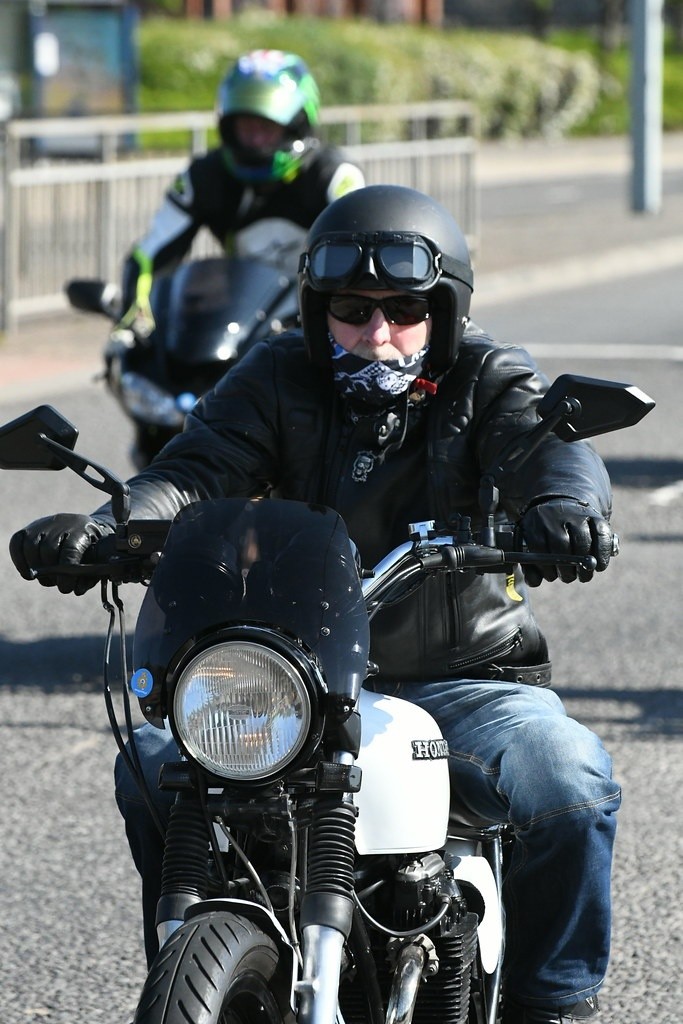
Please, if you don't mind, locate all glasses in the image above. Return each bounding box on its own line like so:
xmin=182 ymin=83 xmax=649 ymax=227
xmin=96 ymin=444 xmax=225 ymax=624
xmin=322 ymin=294 xmax=438 ymax=326
xmin=304 ymin=231 xmax=445 ymax=292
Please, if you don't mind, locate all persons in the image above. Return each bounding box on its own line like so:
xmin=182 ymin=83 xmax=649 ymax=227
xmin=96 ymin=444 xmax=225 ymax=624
xmin=8 ymin=184 xmax=623 ymax=1024
xmin=113 ymin=46 xmax=368 ymax=339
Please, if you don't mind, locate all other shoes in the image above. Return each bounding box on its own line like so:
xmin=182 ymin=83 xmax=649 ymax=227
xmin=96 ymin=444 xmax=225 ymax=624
xmin=499 ymin=1002 xmax=562 ymax=1024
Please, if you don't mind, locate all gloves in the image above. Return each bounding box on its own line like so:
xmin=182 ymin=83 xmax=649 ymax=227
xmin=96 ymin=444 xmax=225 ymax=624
xmin=10 ymin=514 xmax=122 ymax=596
xmin=506 ymin=499 xmax=608 ymax=587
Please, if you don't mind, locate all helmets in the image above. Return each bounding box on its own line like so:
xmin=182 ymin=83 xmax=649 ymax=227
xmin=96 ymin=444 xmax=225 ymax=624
xmin=298 ymin=186 xmax=473 ymax=368
xmin=216 ymin=50 xmax=324 ymax=184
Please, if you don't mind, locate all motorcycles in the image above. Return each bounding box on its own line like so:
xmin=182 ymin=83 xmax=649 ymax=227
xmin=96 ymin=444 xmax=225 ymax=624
xmin=0 ymin=373 xmax=683 ymax=1024
xmin=62 ymin=255 xmax=300 ymax=472
